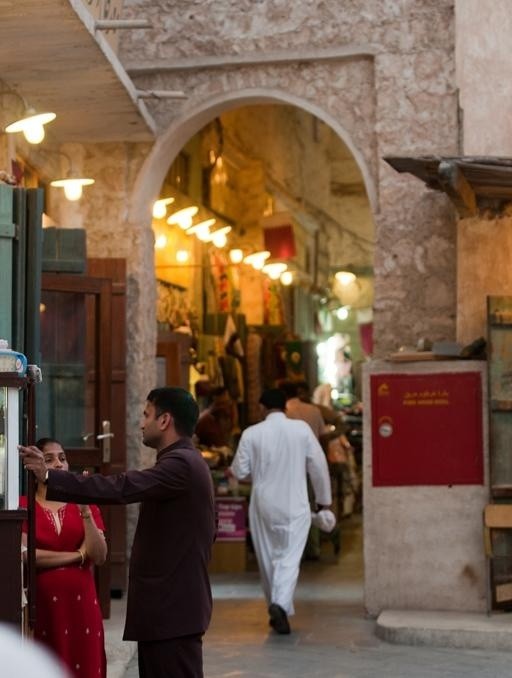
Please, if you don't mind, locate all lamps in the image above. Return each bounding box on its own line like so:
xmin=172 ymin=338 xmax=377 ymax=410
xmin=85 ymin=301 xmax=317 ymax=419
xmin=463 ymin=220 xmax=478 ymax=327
xmin=40 ymin=147 xmax=95 ymax=201
xmin=0 ymin=78 xmax=57 ymax=143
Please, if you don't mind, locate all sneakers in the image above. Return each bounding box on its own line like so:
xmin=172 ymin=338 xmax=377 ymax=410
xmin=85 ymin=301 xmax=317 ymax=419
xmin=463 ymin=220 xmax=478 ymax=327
xmin=267 ymin=602 xmax=293 ymax=636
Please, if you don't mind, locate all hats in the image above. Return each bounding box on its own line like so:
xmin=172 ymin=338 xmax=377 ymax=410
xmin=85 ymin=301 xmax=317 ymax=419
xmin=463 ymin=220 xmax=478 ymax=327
xmin=259 ymin=387 xmax=289 ymax=411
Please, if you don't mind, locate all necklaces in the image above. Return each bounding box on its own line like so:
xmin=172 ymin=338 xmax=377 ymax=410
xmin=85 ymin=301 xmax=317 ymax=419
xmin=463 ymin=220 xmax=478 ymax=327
xmin=36 ymin=494 xmax=66 ymax=533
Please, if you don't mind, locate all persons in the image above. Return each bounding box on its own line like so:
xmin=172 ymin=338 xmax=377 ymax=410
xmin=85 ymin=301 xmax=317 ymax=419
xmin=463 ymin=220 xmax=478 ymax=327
xmin=196 ymin=382 xmax=356 ymax=635
xmin=20 ymin=437 xmax=111 ymax=678
xmin=18 ymin=387 xmax=219 ymax=678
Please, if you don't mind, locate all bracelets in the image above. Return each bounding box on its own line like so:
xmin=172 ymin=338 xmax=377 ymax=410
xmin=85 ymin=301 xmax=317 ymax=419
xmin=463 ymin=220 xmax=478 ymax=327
xmin=80 ymin=508 xmax=92 ymax=520
xmin=77 ymin=549 xmax=84 ymax=565
